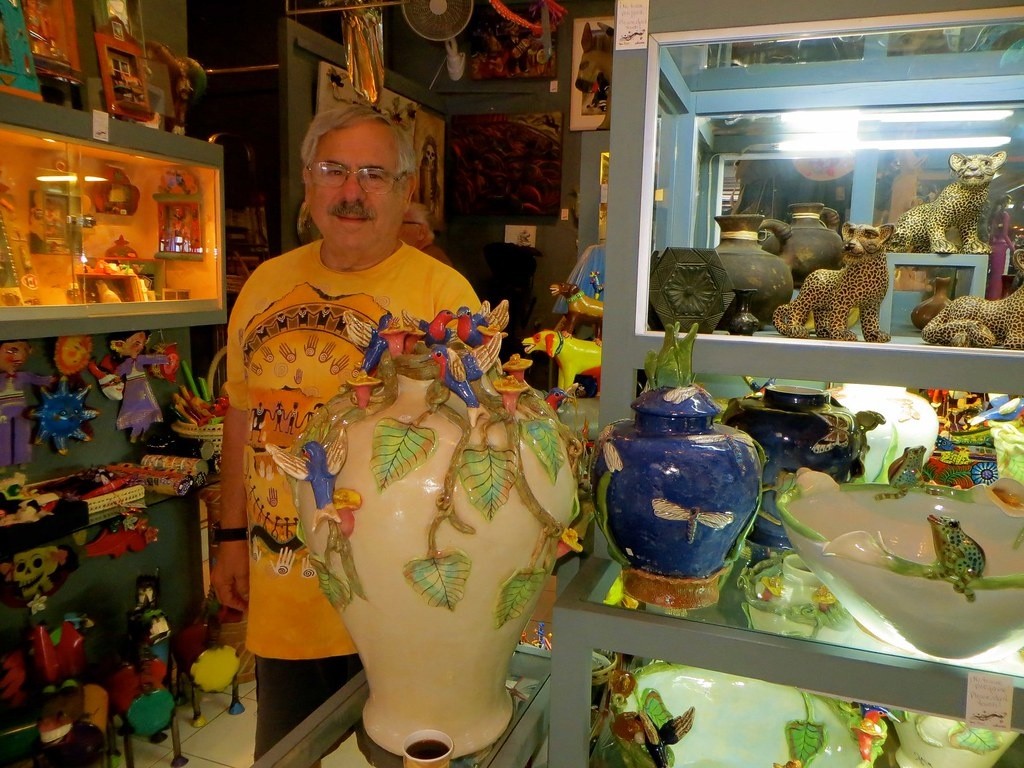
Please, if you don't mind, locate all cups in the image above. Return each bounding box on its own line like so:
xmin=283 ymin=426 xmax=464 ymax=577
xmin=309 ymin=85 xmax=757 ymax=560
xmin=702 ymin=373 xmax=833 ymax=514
xmin=402 ymin=728 xmax=453 ymax=767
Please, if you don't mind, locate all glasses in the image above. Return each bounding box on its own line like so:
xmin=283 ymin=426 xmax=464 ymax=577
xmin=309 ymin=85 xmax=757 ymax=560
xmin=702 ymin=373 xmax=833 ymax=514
xmin=305 ymin=161 xmax=406 ymax=195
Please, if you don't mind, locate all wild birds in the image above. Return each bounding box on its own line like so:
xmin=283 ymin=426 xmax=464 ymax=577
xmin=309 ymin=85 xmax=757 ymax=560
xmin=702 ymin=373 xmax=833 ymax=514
xmin=402 ymin=308 xmax=458 ymax=348
xmin=262 ymin=427 xmax=348 ymax=531
xmin=542 ymin=387 xmax=575 ymax=411
xmin=343 ymin=310 xmax=400 ymax=386
xmin=420 ymin=333 xmax=504 ymax=428
xmin=454 ymin=299 xmax=510 ymax=349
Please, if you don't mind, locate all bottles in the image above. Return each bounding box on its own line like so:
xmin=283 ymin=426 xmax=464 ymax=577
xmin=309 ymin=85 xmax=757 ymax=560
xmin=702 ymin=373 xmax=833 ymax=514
xmin=586 ymin=378 xmax=764 ymax=616
xmin=891 ymin=711 xmax=1015 ymax=768
xmin=266 ymin=302 xmax=590 ymax=756
xmin=721 ymin=383 xmax=867 ymax=550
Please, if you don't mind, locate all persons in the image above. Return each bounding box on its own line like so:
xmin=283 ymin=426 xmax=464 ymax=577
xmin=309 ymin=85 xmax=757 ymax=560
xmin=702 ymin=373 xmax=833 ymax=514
xmin=210 ymin=105 xmax=502 ymax=768
xmin=108 ymin=330 xmax=172 ymax=443
xmin=0 ymin=338 xmax=58 ymax=476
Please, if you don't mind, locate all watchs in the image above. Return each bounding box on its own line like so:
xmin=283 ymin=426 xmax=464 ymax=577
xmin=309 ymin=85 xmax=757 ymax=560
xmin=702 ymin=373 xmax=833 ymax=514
xmin=211 ymin=521 xmax=249 ymax=549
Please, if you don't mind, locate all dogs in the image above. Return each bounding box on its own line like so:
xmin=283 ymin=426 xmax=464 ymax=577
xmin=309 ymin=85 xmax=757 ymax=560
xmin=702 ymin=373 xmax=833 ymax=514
xmin=521 ymin=329 xmax=602 ymax=393
xmin=548 ymin=282 xmax=603 ymax=346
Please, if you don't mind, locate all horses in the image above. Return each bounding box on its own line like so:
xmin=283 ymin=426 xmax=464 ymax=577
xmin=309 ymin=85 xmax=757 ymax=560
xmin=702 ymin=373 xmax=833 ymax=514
xmin=573 ymin=20 xmax=614 ymax=131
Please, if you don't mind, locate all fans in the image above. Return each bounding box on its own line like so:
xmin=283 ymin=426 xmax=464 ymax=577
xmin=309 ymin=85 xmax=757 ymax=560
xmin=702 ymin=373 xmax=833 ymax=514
xmin=403 ymin=0 xmax=474 ymax=82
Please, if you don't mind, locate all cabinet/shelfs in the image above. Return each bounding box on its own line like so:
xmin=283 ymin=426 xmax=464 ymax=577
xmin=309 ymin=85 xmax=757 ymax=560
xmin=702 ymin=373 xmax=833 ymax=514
xmin=0 ymin=91 xmax=228 ymax=342
xmin=547 ymin=1 xmax=1023 ymax=766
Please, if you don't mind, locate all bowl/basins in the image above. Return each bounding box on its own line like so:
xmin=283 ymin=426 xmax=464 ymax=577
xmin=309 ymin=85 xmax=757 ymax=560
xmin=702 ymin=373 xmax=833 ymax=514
xmin=779 ymin=461 xmax=1024 ymax=659
xmin=606 ymin=661 xmax=885 ymax=768
